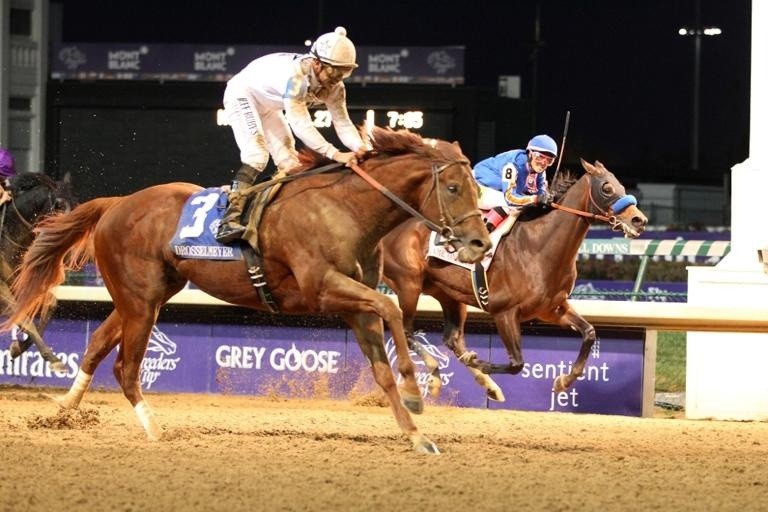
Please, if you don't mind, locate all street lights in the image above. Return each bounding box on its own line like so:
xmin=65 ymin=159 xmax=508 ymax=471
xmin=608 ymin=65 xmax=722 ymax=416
xmin=677 ymin=24 xmax=726 ymax=184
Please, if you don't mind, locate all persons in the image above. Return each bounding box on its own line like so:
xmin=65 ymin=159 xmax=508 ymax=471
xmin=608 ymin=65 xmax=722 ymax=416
xmin=0 ymin=149 xmax=15 ymax=207
xmin=217 ymin=31 xmax=367 ymax=246
xmin=471 ymin=134 xmax=559 ymax=234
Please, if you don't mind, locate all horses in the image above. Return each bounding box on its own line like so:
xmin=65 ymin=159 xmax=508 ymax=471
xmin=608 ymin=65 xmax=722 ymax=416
xmin=378 ymin=157 xmax=650 ymax=403
xmin=0 ymin=121 xmax=492 ymax=455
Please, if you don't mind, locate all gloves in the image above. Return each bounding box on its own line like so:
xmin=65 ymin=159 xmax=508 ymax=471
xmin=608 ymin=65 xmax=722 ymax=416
xmin=540 ymin=190 xmax=558 ymax=205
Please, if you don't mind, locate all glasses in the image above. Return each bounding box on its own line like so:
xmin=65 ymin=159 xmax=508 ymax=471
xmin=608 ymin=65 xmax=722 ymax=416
xmin=532 ymin=152 xmax=556 ymax=167
xmin=326 ymin=66 xmax=354 ymax=80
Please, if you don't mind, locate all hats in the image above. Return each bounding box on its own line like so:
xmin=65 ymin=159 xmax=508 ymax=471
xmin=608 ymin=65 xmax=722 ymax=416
xmin=309 ymin=26 xmax=360 ymax=70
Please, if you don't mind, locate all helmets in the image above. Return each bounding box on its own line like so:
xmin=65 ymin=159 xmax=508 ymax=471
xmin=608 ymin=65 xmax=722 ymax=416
xmin=526 ymin=133 xmax=558 ymax=156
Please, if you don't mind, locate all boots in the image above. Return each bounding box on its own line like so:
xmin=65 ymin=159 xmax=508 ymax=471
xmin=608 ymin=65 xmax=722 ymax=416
xmin=215 ymin=180 xmax=255 ymax=244
